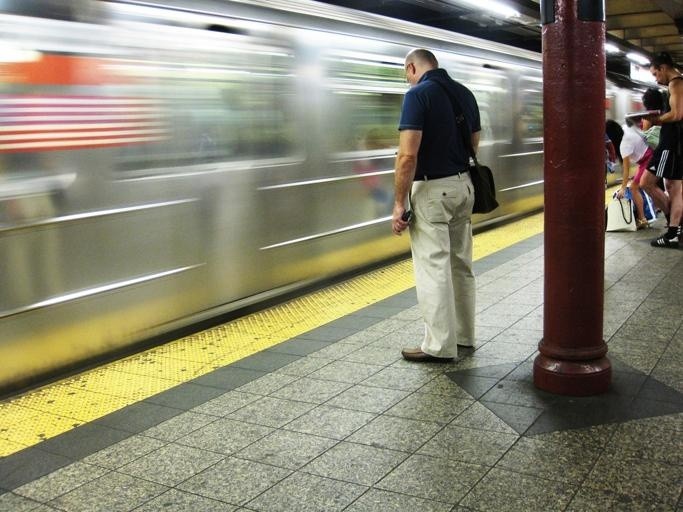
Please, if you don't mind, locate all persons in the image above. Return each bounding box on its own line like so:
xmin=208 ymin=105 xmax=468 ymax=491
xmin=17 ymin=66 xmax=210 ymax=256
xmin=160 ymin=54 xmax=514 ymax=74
xmin=390 ymin=46 xmax=480 ymax=362
xmin=604 ymin=53 xmax=682 ymax=249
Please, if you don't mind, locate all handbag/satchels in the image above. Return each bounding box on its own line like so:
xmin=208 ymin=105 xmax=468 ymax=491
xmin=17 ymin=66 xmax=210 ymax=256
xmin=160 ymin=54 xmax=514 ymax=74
xmin=612 ymin=185 xmax=657 ymax=225
xmin=604 ymin=198 xmax=637 ymax=232
xmin=631 ymin=125 xmax=661 ymax=152
xmin=469 ymin=164 xmax=499 ymax=214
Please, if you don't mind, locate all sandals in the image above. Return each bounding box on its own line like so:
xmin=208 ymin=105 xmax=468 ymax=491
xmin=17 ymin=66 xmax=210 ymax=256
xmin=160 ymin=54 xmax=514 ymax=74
xmin=635 ymin=218 xmax=648 ymax=230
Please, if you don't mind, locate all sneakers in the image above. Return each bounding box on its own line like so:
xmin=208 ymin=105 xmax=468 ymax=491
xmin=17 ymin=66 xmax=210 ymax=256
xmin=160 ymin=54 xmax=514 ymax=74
xmin=650 ymin=232 xmax=678 ymax=248
xmin=665 ymin=214 xmax=682 ymax=243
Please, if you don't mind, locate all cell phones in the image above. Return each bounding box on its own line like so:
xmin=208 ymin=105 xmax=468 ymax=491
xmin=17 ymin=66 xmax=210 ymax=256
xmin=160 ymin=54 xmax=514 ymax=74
xmin=402 ymin=211 xmax=413 ymax=225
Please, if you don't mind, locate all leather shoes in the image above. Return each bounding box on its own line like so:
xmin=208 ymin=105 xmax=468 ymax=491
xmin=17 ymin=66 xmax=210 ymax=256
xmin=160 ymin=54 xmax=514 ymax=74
xmin=401 ymin=348 xmax=452 ymax=362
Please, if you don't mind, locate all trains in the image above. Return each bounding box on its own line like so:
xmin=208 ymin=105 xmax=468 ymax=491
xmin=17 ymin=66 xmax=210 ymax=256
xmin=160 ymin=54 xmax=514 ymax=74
xmin=0 ymin=2 xmax=667 ymax=390
